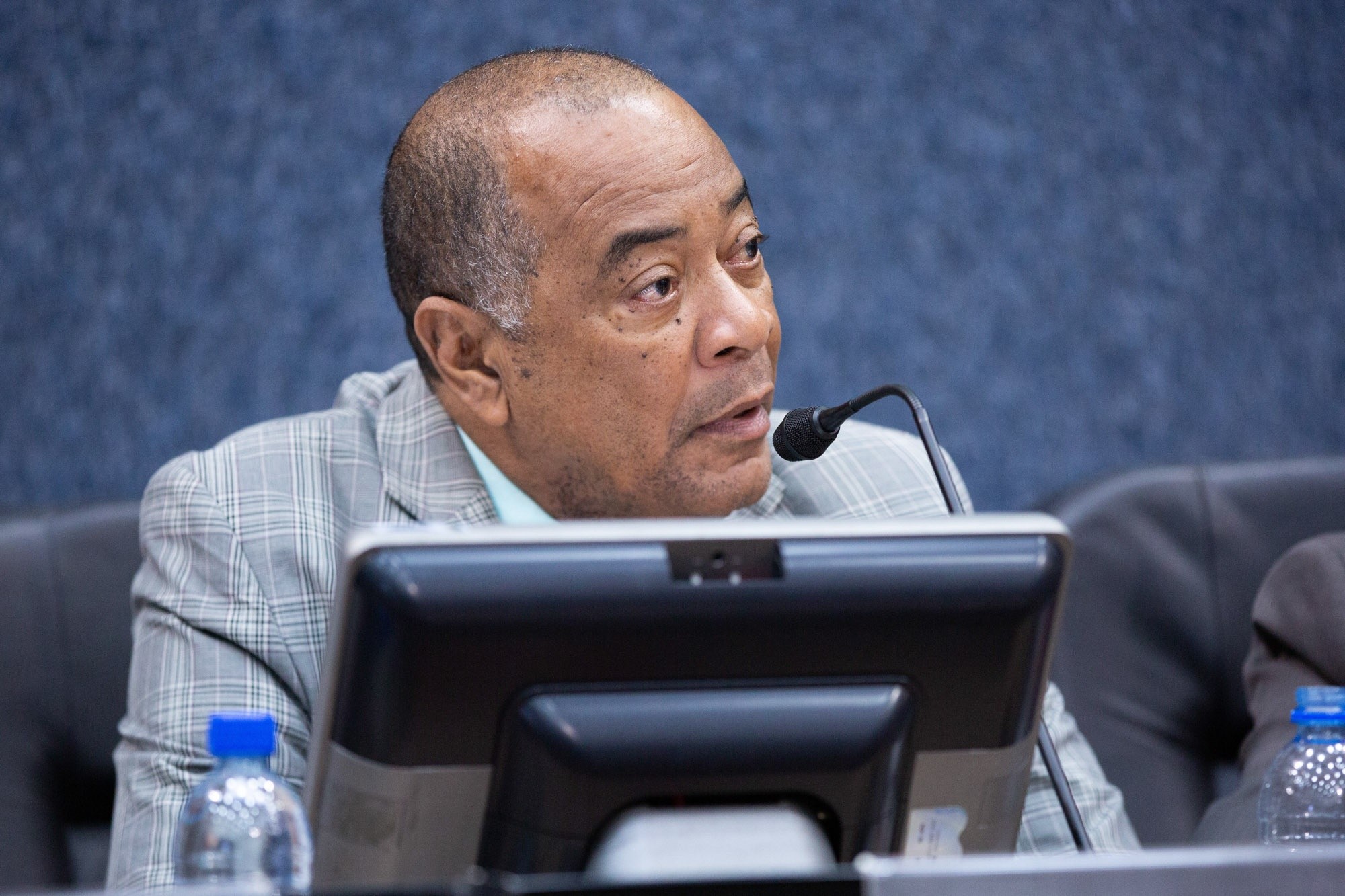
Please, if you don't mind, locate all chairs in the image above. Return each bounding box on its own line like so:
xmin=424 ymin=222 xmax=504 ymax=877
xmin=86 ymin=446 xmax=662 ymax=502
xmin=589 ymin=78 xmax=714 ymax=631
xmin=0 ymin=506 xmax=141 ymax=896
xmin=1029 ymin=455 xmax=1345 ymax=846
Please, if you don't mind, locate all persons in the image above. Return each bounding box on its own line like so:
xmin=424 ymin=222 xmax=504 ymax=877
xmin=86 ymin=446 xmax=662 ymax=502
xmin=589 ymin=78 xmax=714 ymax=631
xmin=1194 ymin=530 xmax=1345 ymax=860
xmin=100 ymin=51 xmax=1152 ymax=896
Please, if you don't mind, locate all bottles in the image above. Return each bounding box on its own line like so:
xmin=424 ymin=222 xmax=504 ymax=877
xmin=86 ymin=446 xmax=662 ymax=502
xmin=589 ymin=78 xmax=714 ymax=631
xmin=171 ymin=712 xmax=313 ymax=896
xmin=1253 ymin=685 xmax=1345 ymax=847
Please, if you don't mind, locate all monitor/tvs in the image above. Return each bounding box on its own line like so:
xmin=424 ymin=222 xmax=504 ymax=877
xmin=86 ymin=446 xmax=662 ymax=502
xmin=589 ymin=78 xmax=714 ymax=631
xmin=304 ymin=511 xmax=1071 ymax=896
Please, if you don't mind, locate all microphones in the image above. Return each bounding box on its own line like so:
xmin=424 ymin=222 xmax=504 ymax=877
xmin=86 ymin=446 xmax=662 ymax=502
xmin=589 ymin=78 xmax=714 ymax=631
xmin=772 ymin=384 xmax=1096 ymax=855
